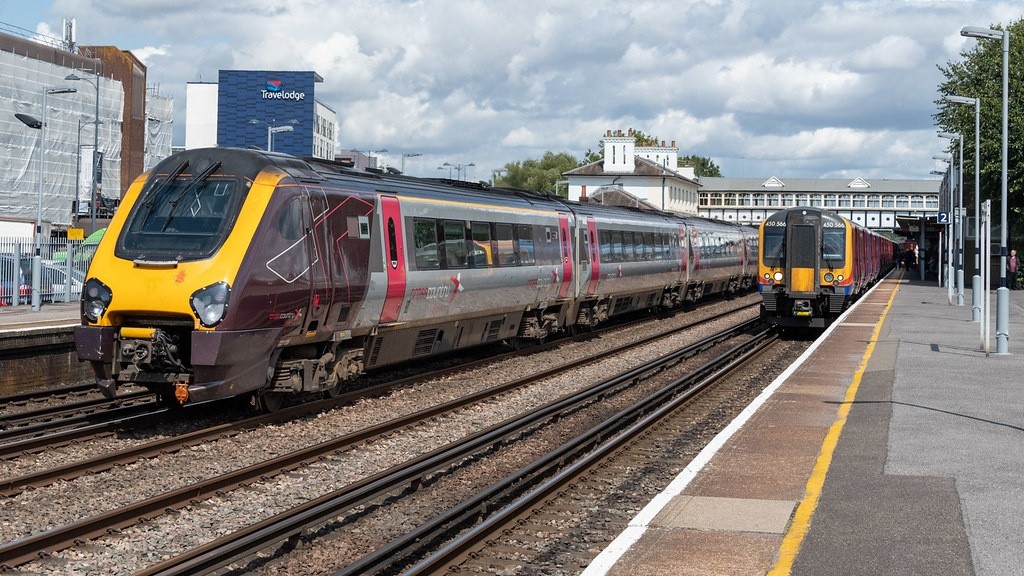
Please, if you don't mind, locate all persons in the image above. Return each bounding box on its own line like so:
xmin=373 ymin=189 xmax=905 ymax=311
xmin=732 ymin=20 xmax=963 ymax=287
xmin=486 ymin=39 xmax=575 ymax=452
xmin=1006 ymin=250 xmax=1021 ymax=291
xmin=896 ymin=246 xmax=938 ymax=273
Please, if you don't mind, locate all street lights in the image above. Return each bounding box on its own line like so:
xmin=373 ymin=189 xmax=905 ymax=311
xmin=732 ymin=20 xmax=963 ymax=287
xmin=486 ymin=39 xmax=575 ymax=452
xmin=930 ymin=170 xmax=951 ymax=213
xmin=267 ymin=125 xmax=294 ymax=152
xmin=958 ymin=25 xmax=1010 ymax=355
xmin=944 ymin=96 xmax=981 ymax=322
xmin=14 ymin=86 xmax=77 ymax=312
xmin=437 ymin=162 xmax=476 ymax=182
xmin=75 ymin=118 xmax=104 ymax=226
xmin=247 ymin=118 xmax=300 ymax=152
xmin=350 ymin=149 xmax=388 ymax=168
xmin=64 ymin=72 xmax=100 ymax=235
xmin=931 ymin=155 xmax=958 ymax=306
xmin=938 ymin=133 xmax=964 ymax=306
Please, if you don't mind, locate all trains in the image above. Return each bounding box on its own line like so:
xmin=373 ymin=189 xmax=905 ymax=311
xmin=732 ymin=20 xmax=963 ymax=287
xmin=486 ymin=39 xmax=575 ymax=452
xmin=73 ymin=146 xmax=761 ymax=420
xmin=757 ymin=206 xmax=899 ymax=329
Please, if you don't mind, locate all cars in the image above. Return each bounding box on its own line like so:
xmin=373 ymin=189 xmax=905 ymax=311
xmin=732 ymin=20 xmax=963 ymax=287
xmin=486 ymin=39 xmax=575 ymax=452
xmin=41 ymin=260 xmax=86 ymax=294
xmin=0 ymin=252 xmax=52 ymax=306
